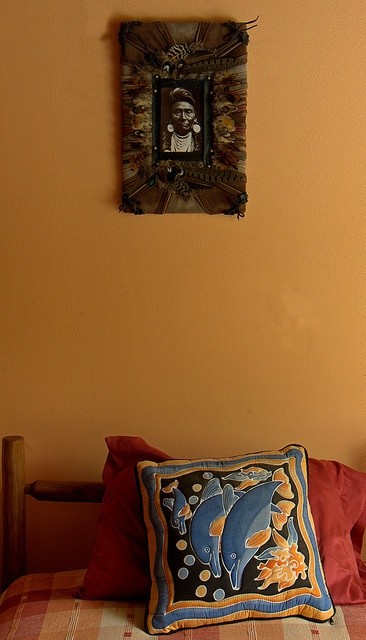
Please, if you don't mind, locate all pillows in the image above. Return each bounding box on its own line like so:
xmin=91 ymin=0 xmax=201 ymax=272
xmin=74 ymin=435 xmax=366 ymax=607
xmin=134 ymin=443 xmax=336 ymax=636
xmin=0 ymin=435 xmax=366 ymax=639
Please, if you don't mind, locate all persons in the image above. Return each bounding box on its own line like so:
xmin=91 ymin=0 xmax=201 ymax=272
xmin=161 ymin=87 xmax=202 ymax=152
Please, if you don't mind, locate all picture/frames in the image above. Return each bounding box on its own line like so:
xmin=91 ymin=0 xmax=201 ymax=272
xmin=115 ymin=14 xmax=261 ymax=220
